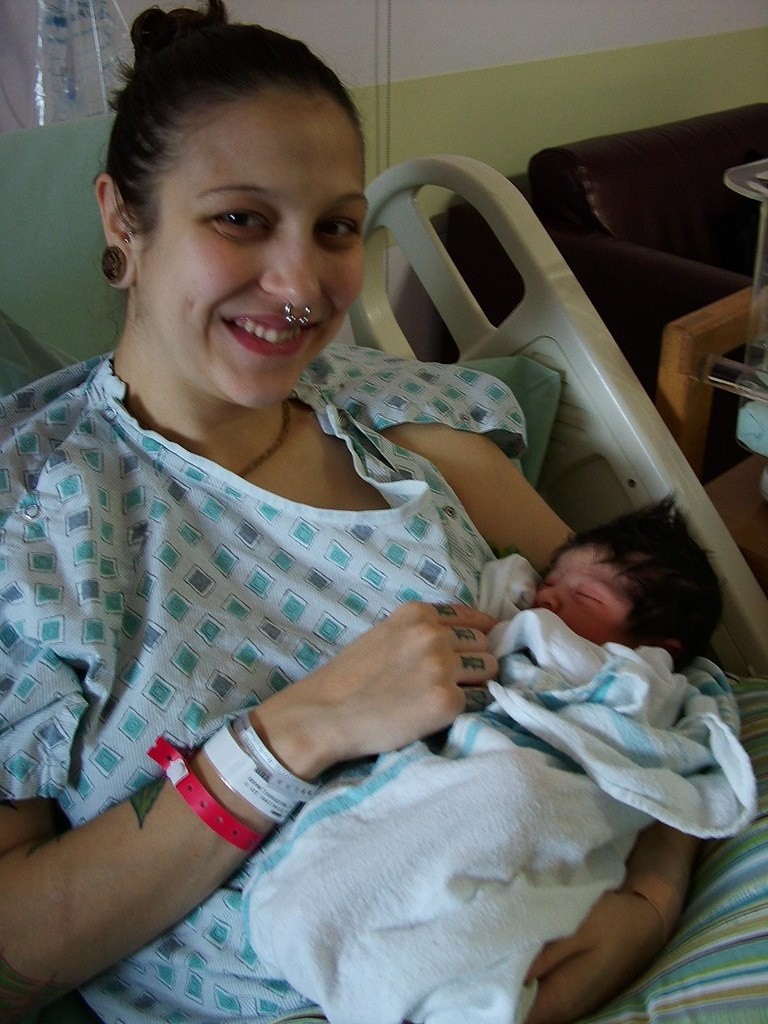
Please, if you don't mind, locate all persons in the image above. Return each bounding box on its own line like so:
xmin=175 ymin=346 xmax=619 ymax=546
xmin=241 ymin=492 xmax=722 ymax=1024
xmin=1 ymin=1 xmax=768 ymax=1024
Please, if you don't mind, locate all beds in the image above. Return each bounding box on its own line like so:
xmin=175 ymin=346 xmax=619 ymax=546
xmin=0 ymin=107 xmax=768 ymax=1024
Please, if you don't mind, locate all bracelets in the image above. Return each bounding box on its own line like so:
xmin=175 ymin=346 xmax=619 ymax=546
xmin=629 ymin=873 xmax=681 ymax=938
xmin=200 ymin=721 xmax=301 ymax=826
xmin=144 ymin=735 xmax=266 ymax=849
xmin=233 ymin=715 xmax=323 ymax=801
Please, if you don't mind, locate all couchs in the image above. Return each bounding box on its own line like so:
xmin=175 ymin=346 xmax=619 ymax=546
xmin=441 ymin=104 xmax=768 ymax=489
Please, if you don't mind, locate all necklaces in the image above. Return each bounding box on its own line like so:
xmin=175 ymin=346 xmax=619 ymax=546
xmin=238 ymin=399 xmax=290 ymax=477
